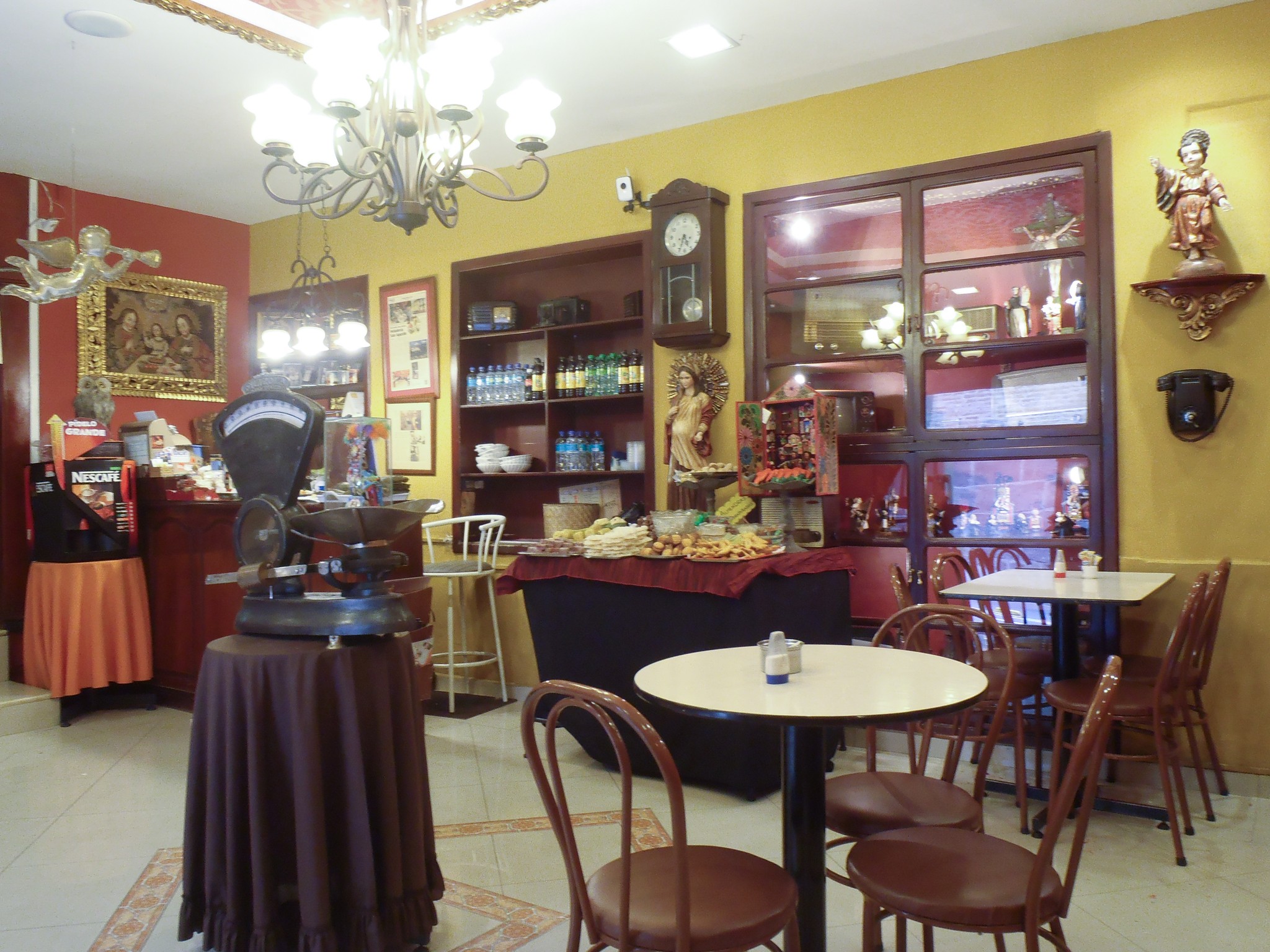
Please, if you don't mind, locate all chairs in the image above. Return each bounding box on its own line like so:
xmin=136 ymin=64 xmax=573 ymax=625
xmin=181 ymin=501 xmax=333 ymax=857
xmin=890 ymin=549 xmax=1231 ymax=866
xmin=827 ymin=605 xmax=1015 ymax=952
xmin=846 ymin=654 xmax=1121 ymax=952
xmin=519 ymin=678 xmax=802 ymax=952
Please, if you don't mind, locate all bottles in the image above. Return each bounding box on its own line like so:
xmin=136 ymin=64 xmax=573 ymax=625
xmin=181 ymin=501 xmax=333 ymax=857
xmin=765 ymin=630 xmax=789 ymax=684
xmin=466 ymin=358 xmax=544 ymax=404
xmin=554 ymin=348 xmax=643 ymax=399
xmin=620 ymin=440 xmax=646 ymax=471
xmin=554 ymin=430 xmax=605 ymax=472
xmin=1053 ymin=549 xmax=1066 ymax=578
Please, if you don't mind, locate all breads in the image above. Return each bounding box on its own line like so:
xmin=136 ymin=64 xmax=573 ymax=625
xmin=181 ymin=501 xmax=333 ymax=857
xmin=637 ymin=514 xmax=782 ymax=559
xmin=694 ymin=462 xmax=738 ymax=472
xmin=525 ymin=516 xmax=627 ymax=555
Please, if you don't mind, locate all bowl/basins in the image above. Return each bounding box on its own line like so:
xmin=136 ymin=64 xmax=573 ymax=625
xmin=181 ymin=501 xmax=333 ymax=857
xmin=1083 ymin=565 xmax=1097 ymax=579
xmin=649 ymin=509 xmax=699 ymax=539
xmin=757 ymin=637 xmax=805 ymax=674
xmin=473 ymin=443 xmax=534 ymax=474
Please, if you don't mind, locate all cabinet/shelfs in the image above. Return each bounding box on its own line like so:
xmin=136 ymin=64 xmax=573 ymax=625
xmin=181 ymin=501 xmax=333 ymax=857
xmin=756 ymin=147 xmax=1101 ymax=634
xmin=250 ymin=274 xmax=369 ymax=484
xmin=451 ymin=229 xmax=655 ymax=554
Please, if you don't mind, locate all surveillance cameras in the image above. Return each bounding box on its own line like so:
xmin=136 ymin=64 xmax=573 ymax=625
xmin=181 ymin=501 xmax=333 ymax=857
xmin=616 ymin=175 xmax=642 ymax=212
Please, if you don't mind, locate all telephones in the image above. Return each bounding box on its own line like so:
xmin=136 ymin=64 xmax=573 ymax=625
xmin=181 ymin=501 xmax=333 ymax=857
xmin=1155 ymin=369 xmax=1235 ymax=443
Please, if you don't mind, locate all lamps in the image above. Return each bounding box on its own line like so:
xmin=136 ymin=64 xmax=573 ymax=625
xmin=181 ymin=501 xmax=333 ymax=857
xmin=256 ymin=171 xmax=369 ymax=362
xmin=240 ymin=1 xmax=559 ymax=235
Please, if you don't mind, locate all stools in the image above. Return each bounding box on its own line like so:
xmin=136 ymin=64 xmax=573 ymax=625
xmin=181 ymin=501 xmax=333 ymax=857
xmin=422 ymin=514 xmax=507 ymax=712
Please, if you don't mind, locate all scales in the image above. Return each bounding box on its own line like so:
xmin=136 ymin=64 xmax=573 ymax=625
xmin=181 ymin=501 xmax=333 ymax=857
xmin=203 ymin=374 xmax=445 ymax=651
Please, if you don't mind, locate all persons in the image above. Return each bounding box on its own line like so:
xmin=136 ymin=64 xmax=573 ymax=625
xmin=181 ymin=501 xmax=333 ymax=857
xmin=663 ymin=363 xmax=718 ymax=515
xmin=1003 ymin=282 xmax=1085 ymax=337
xmin=845 ymin=488 xmax=1082 ymax=539
xmin=767 ymin=446 xmax=816 ymax=473
xmin=1150 ymin=136 xmax=1233 ymax=262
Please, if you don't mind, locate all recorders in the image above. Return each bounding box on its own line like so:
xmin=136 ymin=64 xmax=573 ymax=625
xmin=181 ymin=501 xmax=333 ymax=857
xmin=469 ymin=301 xmax=515 ymax=333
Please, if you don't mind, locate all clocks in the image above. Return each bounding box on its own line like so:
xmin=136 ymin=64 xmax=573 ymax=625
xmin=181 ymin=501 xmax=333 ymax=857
xmin=650 ymin=179 xmax=730 ymax=348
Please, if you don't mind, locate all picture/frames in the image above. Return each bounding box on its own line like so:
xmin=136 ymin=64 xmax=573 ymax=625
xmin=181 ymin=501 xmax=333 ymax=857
xmin=379 ymin=276 xmax=440 ymax=399
xmin=384 ymin=397 xmax=436 ymax=475
xmin=77 ymin=270 xmax=228 ymax=403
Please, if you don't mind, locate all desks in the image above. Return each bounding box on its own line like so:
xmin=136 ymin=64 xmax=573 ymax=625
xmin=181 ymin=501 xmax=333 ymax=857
xmin=940 ymin=567 xmax=1177 ymax=833
xmin=23 ymin=556 xmax=159 ymax=727
xmin=634 ymin=643 xmax=990 ymax=952
xmin=178 ymin=633 xmax=446 ymax=952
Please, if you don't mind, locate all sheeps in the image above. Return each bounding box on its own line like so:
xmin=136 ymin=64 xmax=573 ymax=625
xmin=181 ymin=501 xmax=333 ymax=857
xmin=70 ymin=375 xmax=116 ymax=427
